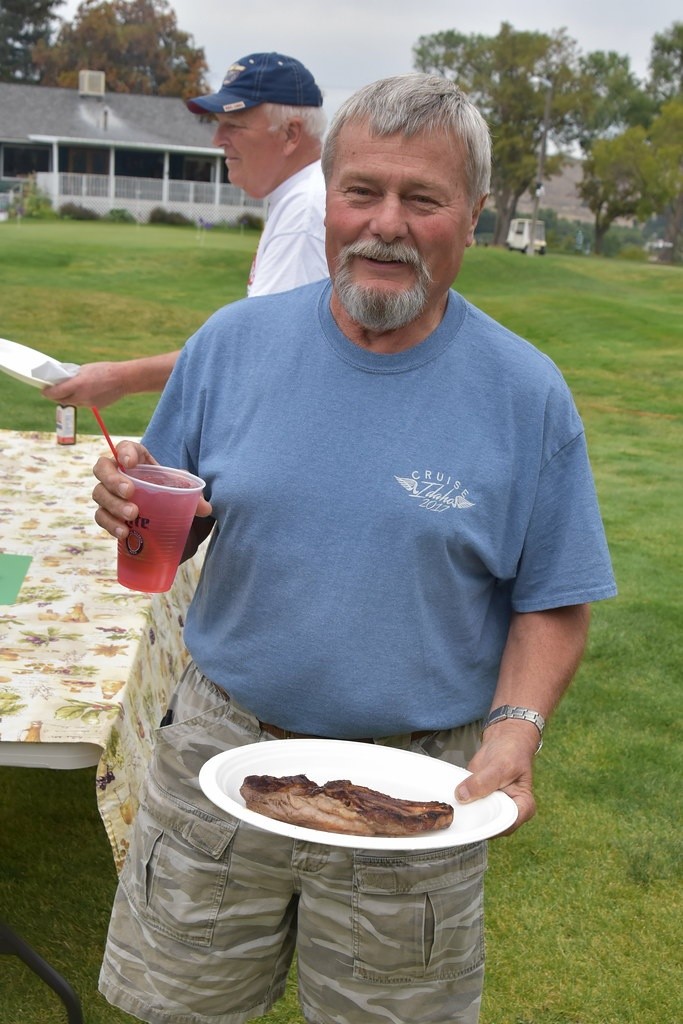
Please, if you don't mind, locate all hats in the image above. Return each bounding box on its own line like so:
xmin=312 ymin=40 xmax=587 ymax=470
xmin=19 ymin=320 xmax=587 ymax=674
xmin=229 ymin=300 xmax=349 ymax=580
xmin=185 ymin=53 xmax=323 ymax=114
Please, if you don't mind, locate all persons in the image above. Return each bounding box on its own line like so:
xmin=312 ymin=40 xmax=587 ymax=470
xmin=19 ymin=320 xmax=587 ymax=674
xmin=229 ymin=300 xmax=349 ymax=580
xmin=92 ymin=71 xmax=617 ymax=1023
xmin=43 ymin=52 xmax=329 ymax=409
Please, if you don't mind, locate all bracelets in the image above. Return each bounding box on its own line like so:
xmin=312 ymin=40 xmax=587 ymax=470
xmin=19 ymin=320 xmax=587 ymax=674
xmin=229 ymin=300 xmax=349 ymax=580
xmin=479 ymin=704 xmax=546 ymax=755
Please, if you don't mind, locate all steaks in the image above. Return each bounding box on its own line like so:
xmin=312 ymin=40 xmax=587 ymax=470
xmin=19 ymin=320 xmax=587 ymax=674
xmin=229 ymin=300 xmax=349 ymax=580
xmin=243 ymin=776 xmax=454 ymax=835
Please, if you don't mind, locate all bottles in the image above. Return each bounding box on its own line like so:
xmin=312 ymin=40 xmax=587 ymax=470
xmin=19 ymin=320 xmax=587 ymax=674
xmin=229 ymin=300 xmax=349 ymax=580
xmin=56 ymin=403 xmax=77 ymax=445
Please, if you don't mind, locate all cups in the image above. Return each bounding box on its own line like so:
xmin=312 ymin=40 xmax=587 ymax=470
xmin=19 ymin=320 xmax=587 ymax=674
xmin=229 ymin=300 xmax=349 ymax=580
xmin=117 ymin=465 xmax=206 ymax=592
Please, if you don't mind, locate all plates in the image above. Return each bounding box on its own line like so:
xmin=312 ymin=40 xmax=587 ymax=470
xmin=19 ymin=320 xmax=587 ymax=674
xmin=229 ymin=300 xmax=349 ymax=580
xmin=0 ymin=338 xmax=71 ymax=389
xmin=198 ymin=740 xmax=518 ymax=851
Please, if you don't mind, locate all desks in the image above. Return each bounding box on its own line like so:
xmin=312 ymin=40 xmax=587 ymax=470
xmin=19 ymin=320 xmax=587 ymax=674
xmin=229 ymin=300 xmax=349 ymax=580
xmin=0 ymin=424 xmax=212 ymax=1024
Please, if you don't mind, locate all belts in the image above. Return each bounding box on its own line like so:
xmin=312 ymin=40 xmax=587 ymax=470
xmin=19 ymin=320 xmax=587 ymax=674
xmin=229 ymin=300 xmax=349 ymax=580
xmin=212 ymin=683 xmax=439 ymax=747
